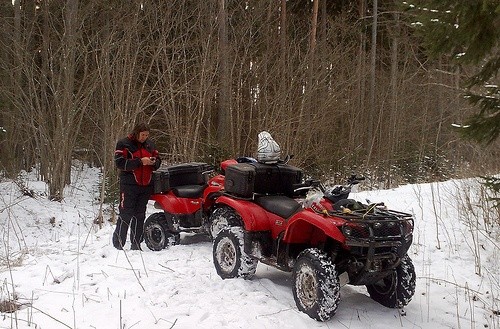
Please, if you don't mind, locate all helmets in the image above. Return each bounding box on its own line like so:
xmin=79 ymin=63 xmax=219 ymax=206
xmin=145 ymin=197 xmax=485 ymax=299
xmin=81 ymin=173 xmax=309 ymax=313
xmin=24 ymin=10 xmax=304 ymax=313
xmin=258 ymin=131 xmax=280 ymax=164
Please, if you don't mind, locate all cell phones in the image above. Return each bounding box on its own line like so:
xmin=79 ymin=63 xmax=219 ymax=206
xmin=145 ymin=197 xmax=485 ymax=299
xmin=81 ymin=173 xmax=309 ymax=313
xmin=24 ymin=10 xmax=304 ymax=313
xmin=149 ymin=156 xmax=156 ymax=162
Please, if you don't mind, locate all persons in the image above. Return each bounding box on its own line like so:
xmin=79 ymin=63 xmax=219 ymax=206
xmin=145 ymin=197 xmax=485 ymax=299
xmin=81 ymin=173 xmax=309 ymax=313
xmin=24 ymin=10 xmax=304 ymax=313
xmin=113 ymin=122 xmax=162 ymax=251
